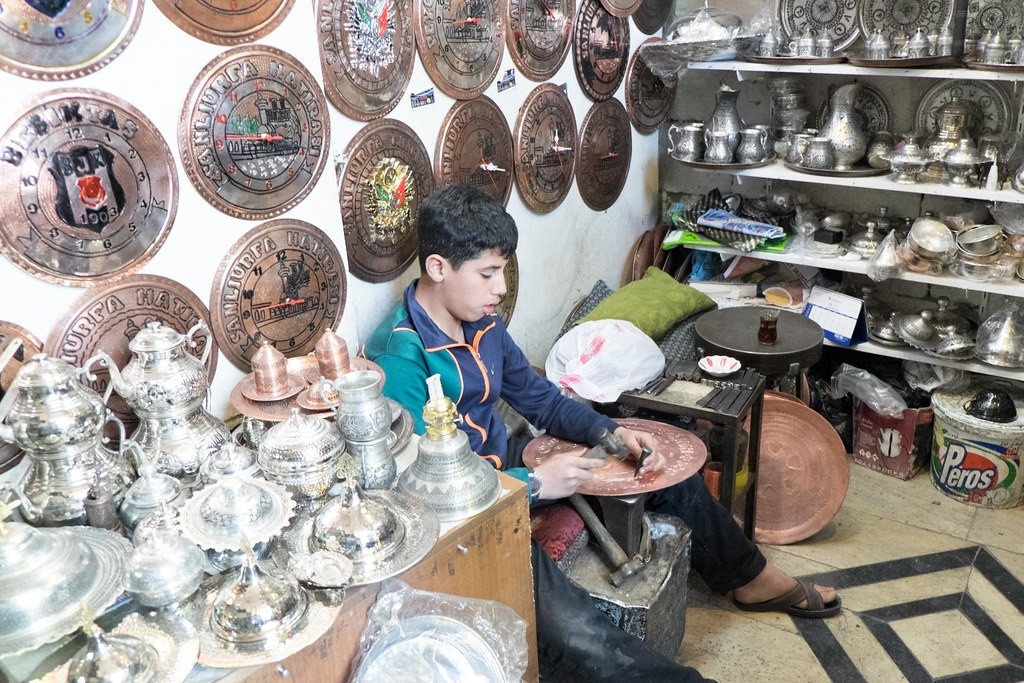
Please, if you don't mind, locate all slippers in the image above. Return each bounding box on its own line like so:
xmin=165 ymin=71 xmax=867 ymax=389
xmin=733 ymin=577 xmax=841 ymax=618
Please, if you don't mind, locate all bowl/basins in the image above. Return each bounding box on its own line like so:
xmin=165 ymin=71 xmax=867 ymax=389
xmin=880 ymin=138 xmax=933 ymax=185
xmin=941 ymin=139 xmax=985 ymax=188
xmin=963 ymin=388 xmax=1017 ymax=420
xmin=698 ymin=355 xmax=741 ymax=376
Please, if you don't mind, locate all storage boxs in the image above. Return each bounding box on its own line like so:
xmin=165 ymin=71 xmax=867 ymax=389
xmin=852 ymin=395 xmax=934 ymax=479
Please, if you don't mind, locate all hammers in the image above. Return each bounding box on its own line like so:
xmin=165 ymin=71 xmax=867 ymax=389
xmin=572 ymin=495 xmax=646 ymax=588
xmin=580 ymin=427 xmax=631 ymax=463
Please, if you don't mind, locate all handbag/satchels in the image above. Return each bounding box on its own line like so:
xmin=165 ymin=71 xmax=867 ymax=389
xmin=544 ymin=318 xmax=665 ymax=408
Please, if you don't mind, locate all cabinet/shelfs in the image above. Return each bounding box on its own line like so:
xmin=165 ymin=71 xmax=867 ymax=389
xmin=655 ymin=58 xmax=1024 ymax=387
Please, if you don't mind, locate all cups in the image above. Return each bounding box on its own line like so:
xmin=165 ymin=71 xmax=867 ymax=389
xmin=320 ymin=370 xmax=397 ymax=489
xmin=307 ymin=328 xmax=350 ymax=381
xmin=759 ymin=25 xmax=1024 ymax=64
xmin=758 ymin=309 xmax=781 ymax=345
xmin=250 ymin=341 xmax=289 ymax=397
xmin=900 ymin=219 xmax=1024 ymax=283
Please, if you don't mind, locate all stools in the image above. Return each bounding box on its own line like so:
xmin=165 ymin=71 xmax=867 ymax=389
xmin=629 ymin=370 xmax=767 ymax=542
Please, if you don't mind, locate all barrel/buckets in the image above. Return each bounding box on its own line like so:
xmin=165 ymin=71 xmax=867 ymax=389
xmin=929 ymin=375 xmax=1024 ymax=509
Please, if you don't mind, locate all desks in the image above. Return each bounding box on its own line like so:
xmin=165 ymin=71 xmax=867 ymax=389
xmin=229 ymin=434 xmax=536 ymax=683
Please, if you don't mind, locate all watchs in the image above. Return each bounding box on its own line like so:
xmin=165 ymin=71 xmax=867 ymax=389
xmin=528 ymin=466 xmax=540 ymax=502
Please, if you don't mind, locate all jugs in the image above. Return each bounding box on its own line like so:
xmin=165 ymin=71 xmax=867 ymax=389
xmin=667 ymin=79 xmax=866 ymax=178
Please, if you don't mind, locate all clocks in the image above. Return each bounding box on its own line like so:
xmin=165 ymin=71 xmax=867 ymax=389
xmin=0 ymin=0 xmax=707 ymax=496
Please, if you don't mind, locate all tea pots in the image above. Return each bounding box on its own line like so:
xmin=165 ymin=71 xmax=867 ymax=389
xmin=0 ymin=319 xmax=230 ymax=530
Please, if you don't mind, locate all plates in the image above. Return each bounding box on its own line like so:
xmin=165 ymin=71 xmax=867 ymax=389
xmin=297 ymin=388 xmax=340 ymax=410
xmin=241 ymin=373 xmax=306 ymax=401
xmin=307 ymin=357 xmax=367 ymax=383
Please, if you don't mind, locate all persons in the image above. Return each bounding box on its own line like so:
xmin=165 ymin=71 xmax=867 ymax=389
xmin=356 ymin=185 xmax=842 ymax=683
